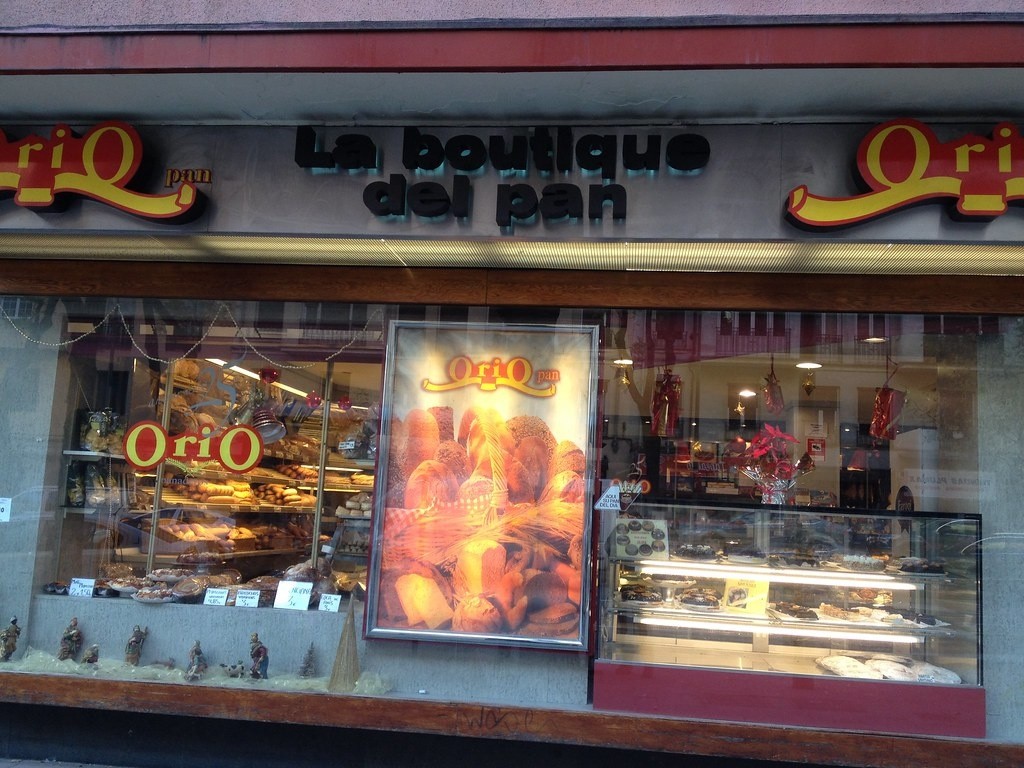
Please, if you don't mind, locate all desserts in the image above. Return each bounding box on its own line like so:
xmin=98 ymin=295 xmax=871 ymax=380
xmin=774 ymin=599 xmax=936 ymax=632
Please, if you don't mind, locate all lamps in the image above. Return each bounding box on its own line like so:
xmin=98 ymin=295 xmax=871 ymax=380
xmin=634 ymin=559 xmax=925 ymax=591
xmin=634 ymin=617 xmax=924 ymax=643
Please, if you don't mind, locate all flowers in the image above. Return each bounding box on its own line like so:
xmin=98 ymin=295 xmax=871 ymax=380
xmin=721 ymin=423 xmax=816 ymax=505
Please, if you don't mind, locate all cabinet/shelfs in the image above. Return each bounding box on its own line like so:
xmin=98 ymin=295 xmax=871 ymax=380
xmin=58 ymin=447 xmax=375 ymax=570
xmin=593 ymin=478 xmax=987 ymax=743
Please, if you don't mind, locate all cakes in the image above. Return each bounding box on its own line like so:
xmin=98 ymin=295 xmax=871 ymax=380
xmin=621 ymin=587 xmax=719 ymax=608
xmin=674 ymin=543 xmax=946 ymax=575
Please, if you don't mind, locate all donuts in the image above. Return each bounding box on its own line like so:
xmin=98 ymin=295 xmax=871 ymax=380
xmin=616 ymin=519 xmax=666 ymax=556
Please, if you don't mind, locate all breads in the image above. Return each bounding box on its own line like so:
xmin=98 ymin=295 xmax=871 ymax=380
xmin=378 ymin=406 xmax=586 ymax=638
xmin=44 ymin=361 xmax=377 ymax=613
